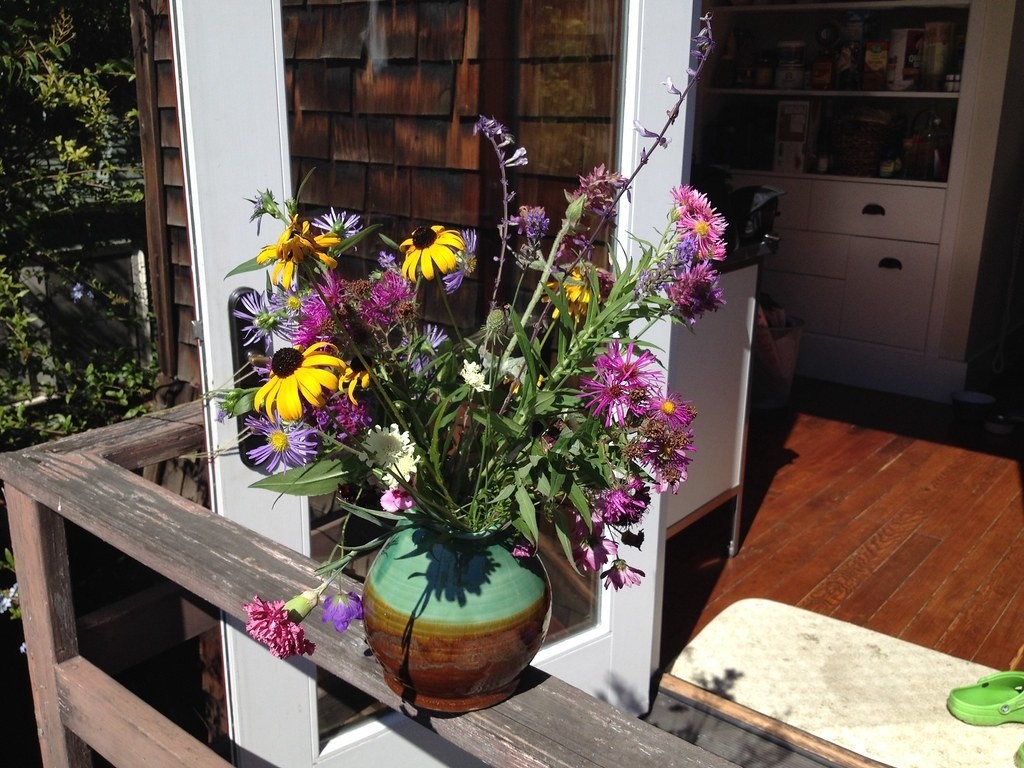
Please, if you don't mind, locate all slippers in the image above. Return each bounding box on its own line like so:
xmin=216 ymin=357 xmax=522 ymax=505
xmin=945 ymin=670 xmax=1024 ymax=729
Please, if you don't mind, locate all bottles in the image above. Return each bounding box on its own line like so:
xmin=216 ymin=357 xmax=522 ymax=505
xmin=721 ymin=31 xmax=736 ymax=88
xmin=917 ymin=104 xmax=937 ymax=180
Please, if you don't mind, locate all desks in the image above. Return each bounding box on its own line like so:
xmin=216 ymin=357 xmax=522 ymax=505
xmin=666 ymin=234 xmax=778 ymax=558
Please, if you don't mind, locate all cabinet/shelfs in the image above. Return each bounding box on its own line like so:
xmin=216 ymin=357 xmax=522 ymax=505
xmin=689 ymin=0 xmax=1016 ymax=401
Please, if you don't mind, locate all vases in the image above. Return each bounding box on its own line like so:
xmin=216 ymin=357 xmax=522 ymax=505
xmin=361 ymin=509 xmax=551 ymax=713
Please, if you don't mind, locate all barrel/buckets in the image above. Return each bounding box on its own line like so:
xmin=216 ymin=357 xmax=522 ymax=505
xmin=752 ymin=315 xmax=804 ymax=409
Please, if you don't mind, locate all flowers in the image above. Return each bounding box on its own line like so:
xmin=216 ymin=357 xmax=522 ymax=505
xmin=178 ymin=12 xmax=728 ymax=658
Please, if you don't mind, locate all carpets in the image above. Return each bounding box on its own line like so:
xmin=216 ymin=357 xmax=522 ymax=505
xmin=664 ymin=598 xmax=1024 ymax=768
